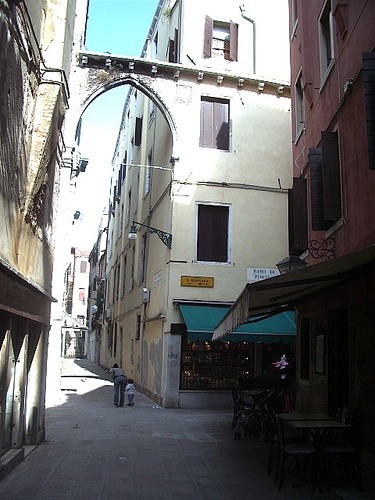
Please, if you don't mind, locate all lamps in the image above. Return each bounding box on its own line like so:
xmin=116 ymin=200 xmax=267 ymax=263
xmin=127 ymin=218 xmax=172 ymax=249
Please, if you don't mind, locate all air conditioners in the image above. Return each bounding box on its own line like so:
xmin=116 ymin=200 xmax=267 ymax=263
xmin=104 ymin=308 xmax=111 ymax=318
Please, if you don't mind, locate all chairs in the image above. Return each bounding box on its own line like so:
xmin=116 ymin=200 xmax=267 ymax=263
xmin=313 ymin=408 xmax=367 ymax=495
xmin=230 ymin=381 xmax=281 ymax=439
xmin=270 ymin=411 xmax=318 ymax=492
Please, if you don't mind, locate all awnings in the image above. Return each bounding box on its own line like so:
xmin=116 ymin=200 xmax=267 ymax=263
xmin=179 ymin=304 xmax=298 ymax=343
xmin=210 ymin=243 xmax=375 ymax=342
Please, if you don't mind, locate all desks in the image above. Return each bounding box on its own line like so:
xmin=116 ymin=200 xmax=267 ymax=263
xmin=286 ymin=420 xmax=354 ymax=490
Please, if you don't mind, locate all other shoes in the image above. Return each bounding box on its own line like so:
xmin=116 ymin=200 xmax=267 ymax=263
xmin=120 ymin=403 xmax=123 ymax=405
xmin=113 ymin=403 xmax=118 ymax=405
xmin=128 ymin=403 xmax=134 ymax=405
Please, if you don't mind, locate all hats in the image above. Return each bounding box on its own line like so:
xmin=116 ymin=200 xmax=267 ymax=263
xmin=113 ymin=363 xmax=119 ymax=368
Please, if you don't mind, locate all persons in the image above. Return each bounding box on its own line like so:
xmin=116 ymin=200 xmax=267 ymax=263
xmin=110 ymin=363 xmax=126 ymax=407
xmin=126 ymin=378 xmax=136 ymax=406
